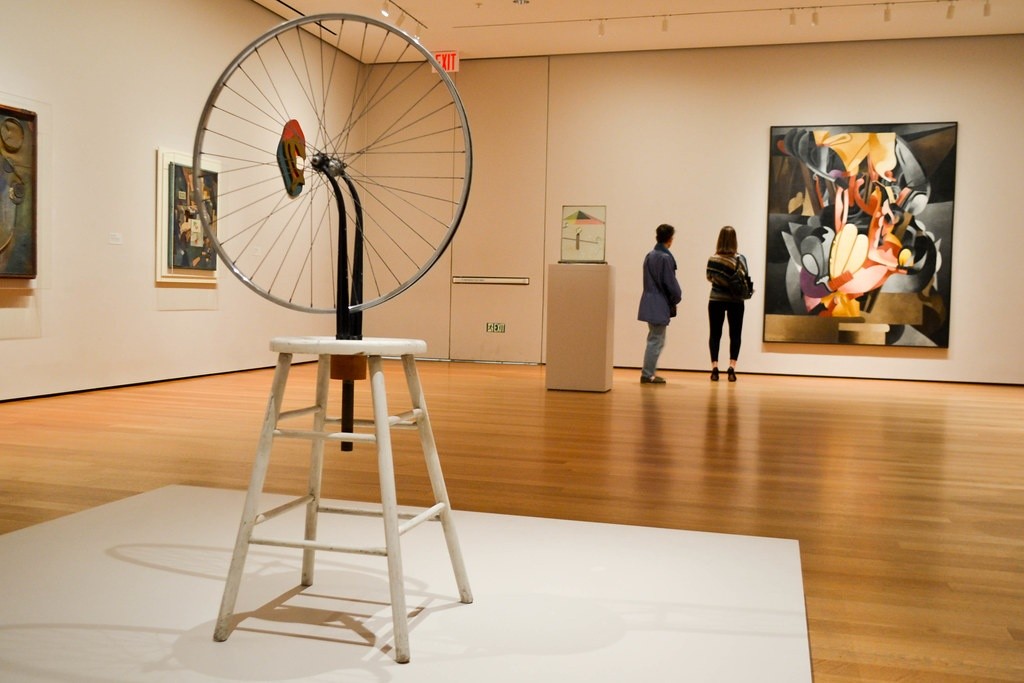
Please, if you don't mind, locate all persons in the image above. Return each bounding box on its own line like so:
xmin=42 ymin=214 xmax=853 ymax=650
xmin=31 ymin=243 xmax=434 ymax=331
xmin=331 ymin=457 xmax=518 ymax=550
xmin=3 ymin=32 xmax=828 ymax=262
xmin=638 ymin=224 xmax=682 ymax=384
xmin=706 ymin=226 xmax=749 ymax=381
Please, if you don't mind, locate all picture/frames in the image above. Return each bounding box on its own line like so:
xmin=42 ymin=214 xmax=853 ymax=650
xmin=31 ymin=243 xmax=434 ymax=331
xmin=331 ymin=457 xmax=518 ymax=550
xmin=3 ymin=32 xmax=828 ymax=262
xmin=559 ymin=204 xmax=607 ymax=264
xmin=0 ymin=102 xmax=38 ymax=279
xmin=762 ymin=121 xmax=958 ymax=349
xmin=155 ymin=146 xmax=222 ymax=283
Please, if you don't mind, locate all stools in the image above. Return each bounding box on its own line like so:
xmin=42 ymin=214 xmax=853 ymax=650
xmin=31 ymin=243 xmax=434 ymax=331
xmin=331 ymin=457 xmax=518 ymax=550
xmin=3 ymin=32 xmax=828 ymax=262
xmin=213 ymin=336 xmax=474 ymax=664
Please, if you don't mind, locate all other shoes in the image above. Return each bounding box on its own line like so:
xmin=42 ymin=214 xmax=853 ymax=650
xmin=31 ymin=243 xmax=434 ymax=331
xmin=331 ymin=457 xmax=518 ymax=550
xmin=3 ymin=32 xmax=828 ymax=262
xmin=640 ymin=374 xmax=666 ymax=384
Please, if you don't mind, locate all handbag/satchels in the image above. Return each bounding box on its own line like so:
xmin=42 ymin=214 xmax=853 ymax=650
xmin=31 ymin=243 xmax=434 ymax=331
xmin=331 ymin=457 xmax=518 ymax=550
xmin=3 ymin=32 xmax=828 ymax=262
xmin=730 ymin=255 xmax=754 ymax=299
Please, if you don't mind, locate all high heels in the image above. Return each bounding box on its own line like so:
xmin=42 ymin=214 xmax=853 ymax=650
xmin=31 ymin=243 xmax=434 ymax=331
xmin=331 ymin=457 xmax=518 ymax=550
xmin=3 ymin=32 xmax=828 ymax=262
xmin=727 ymin=366 xmax=737 ymax=382
xmin=711 ymin=367 xmax=719 ymax=381
